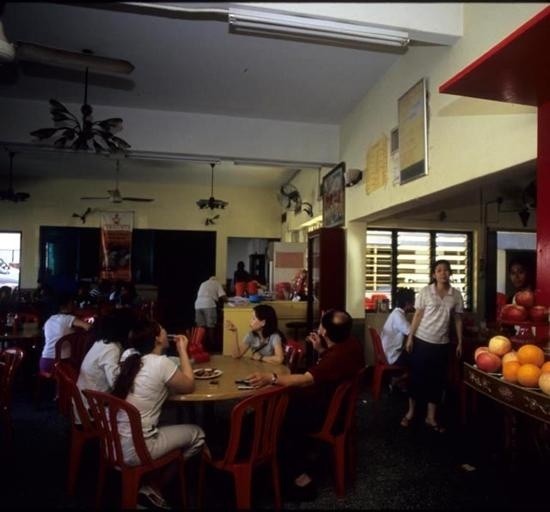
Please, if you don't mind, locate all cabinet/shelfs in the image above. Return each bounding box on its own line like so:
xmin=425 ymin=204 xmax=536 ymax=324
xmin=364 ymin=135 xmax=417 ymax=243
xmin=223 ymin=301 xmax=307 ymax=359
xmin=308 ymin=227 xmax=347 ymax=334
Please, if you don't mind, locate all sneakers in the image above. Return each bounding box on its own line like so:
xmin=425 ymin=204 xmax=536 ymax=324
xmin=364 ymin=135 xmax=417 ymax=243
xmin=137 ymin=486 xmax=169 ymax=509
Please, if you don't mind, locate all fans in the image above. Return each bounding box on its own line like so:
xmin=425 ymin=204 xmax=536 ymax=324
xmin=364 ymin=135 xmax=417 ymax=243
xmin=195 ymin=162 xmax=230 ymax=209
xmin=80 ymin=158 xmax=156 ymax=204
xmin=276 ymin=182 xmax=314 ymax=218
xmin=0 ymin=15 xmax=136 ymax=76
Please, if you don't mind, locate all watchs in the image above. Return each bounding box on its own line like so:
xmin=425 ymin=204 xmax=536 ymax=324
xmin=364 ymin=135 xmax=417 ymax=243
xmin=271 ymin=372 xmax=279 ymax=387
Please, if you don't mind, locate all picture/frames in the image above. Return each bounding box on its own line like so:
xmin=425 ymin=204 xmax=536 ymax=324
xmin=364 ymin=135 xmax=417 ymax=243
xmin=322 ymin=161 xmax=346 ymax=229
xmin=397 ymin=75 xmax=429 ymax=185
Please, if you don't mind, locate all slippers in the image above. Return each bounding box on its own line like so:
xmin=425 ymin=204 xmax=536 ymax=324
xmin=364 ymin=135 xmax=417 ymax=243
xmin=425 ymin=418 xmax=445 ymax=434
xmin=401 ymin=414 xmax=413 ymax=427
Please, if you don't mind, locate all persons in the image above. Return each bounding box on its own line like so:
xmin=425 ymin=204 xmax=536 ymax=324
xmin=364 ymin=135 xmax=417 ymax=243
xmin=70 ymin=278 xmax=143 ymax=309
xmin=379 ymin=288 xmax=418 ymax=391
xmin=247 ymin=310 xmax=364 ymax=501
xmin=31 ymin=276 xmax=51 ymax=305
xmin=195 ymin=276 xmax=228 ymax=353
xmin=98 ymin=317 xmax=206 ymax=512
xmin=71 ymin=310 xmax=137 ymax=427
xmin=0 ymin=286 xmax=13 ymax=312
xmin=40 ymin=294 xmax=93 ymax=401
xmin=400 ymin=260 xmax=464 ymax=434
xmin=508 ymin=260 xmax=536 ymax=306
xmin=231 ymin=260 xmax=251 ymax=291
xmin=225 ymin=305 xmax=284 ymax=364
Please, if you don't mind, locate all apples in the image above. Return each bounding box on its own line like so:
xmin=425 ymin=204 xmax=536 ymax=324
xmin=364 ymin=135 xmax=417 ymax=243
xmin=476 ymin=353 xmax=500 ymax=372
xmin=475 ymin=346 xmax=488 ymax=362
xmin=502 ymin=352 xmax=518 ymax=364
xmin=488 ymin=337 xmax=511 ymax=356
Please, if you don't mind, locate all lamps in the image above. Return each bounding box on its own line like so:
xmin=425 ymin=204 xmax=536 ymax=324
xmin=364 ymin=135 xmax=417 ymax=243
xmin=227 ymin=9 xmax=409 ymax=50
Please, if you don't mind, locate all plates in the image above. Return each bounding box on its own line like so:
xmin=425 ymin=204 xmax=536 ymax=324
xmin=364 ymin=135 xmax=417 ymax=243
xmin=473 ymin=363 xmax=502 ymax=377
xmin=500 ymin=376 xmax=541 ymax=391
xmin=192 ymin=367 xmax=223 ymax=380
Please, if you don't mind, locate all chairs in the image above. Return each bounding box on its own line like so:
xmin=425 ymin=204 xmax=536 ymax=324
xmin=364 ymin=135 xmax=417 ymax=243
xmin=310 ymin=380 xmax=359 ymax=503
xmin=80 ymin=388 xmax=186 ymax=511
xmin=368 ymin=327 xmax=398 ymax=398
xmin=211 ymin=385 xmax=291 ymax=511
xmin=0 ymin=348 xmax=23 ymax=410
xmin=282 ymin=336 xmax=308 ymax=375
xmin=37 ymin=332 xmax=89 ymax=380
xmin=53 ymin=358 xmax=102 ymax=495
